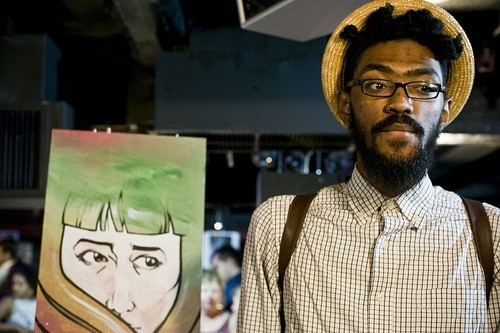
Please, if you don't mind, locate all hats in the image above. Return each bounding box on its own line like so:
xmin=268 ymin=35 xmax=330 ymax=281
xmin=321 ymin=0 xmax=475 ymax=132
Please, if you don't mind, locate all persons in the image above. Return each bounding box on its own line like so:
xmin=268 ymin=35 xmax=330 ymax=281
xmin=0 ymin=230 xmax=242 ymax=333
xmin=234 ymin=0 xmax=499 ymax=333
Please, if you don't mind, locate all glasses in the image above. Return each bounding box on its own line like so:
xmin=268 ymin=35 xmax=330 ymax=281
xmin=346 ymin=79 xmax=446 ymax=99
